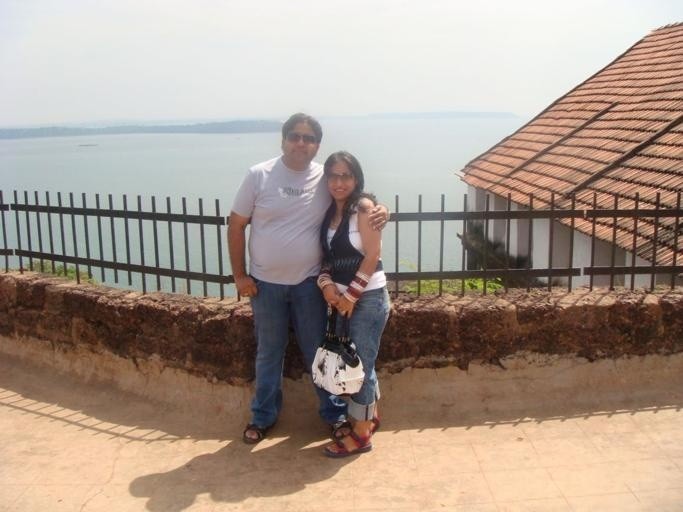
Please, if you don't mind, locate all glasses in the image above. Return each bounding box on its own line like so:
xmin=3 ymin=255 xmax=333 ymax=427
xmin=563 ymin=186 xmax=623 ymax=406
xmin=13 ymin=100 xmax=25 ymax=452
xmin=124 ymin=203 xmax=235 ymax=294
xmin=326 ymin=171 xmax=355 ymax=183
xmin=285 ymin=133 xmax=316 ymax=145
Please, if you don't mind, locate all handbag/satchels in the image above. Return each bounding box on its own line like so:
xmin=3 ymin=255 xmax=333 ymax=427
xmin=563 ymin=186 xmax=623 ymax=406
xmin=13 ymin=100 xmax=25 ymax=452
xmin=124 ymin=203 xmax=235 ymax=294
xmin=311 ymin=342 xmax=366 ymax=396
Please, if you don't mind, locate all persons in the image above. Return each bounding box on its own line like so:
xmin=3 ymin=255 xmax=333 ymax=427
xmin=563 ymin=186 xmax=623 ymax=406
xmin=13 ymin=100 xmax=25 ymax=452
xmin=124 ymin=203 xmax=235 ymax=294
xmin=228 ymin=112 xmax=389 ymax=443
xmin=318 ymin=150 xmax=390 ymax=458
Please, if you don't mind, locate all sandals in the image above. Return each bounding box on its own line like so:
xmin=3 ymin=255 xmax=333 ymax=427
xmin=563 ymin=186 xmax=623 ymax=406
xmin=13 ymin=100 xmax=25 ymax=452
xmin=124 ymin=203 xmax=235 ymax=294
xmin=322 ymin=416 xmax=380 ymax=458
xmin=243 ymin=418 xmax=278 ymax=444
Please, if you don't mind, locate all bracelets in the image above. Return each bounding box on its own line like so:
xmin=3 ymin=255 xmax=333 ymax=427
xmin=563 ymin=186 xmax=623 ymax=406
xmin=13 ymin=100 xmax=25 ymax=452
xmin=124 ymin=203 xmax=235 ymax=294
xmin=343 ymin=271 xmax=370 ymax=303
xmin=316 ymin=270 xmax=335 ymax=289
